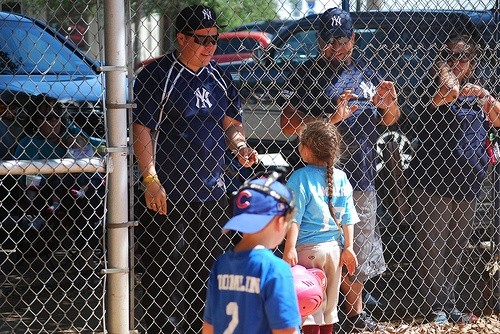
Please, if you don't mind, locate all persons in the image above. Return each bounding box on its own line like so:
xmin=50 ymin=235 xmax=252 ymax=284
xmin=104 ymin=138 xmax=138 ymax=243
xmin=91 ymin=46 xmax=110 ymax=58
xmin=132 ymin=5 xmax=259 ymax=334
xmin=282 ymin=121 xmax=359 ymax=334
xmin=14 ymin=94 xmax=105 ymax=300
xmin=202 ymin=178 xmax=302 ymax=334
xmin=280 ymin=7 xmax=401 ymax=331
xmin=409 ymin=33 xmax=500 ymax=324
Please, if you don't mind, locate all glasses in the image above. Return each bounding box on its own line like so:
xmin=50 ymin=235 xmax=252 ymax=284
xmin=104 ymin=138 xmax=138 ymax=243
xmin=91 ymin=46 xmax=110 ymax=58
xmin=441 ymin=50 xmax=471 ymax=63
xmin=182 ymin=30 xmax=219 ymax=44
xmin=37 ymin=112 xmax=62 ymax=120
xmin=321 ymin=36 xmax=351 ymax=45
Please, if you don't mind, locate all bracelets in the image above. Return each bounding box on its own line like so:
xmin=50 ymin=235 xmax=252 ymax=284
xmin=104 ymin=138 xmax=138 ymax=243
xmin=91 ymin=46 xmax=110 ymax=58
xmin=143 ymin=174 xmax=159 ymax=188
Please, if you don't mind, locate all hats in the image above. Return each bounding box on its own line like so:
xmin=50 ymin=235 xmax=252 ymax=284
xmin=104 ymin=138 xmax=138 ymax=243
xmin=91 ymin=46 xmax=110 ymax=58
xmin=320 ymin=6 xmax=353 ymax=38
xmin=176 ymin=4 xmax=228 ymax=35
xmin=222 ymin=177 xmax=291 ymax=234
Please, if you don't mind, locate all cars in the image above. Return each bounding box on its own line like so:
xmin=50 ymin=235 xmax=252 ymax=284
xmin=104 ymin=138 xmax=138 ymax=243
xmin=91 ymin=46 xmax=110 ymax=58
xmin=1 ymin=11 xmax=149 ymax=124
xmin=230 ymin=18 xmax=301 ymax=32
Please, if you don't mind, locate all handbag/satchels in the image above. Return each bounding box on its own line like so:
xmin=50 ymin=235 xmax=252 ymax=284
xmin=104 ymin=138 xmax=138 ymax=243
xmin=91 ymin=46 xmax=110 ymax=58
xmin=486 ymin=95 xmax=500 ymax=164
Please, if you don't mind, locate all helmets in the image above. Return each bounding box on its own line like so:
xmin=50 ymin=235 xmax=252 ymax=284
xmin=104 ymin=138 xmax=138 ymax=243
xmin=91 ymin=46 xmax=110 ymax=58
xmin=292 ymin=265 xmax=327 ymax=316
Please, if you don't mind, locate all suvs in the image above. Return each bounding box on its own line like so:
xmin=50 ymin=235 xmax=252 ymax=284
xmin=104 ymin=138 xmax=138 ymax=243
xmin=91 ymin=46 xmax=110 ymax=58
xmin=138 ymin=30 xmax=273 ymax=66
xmin=217 ymin=7 xmax=499 ymax=101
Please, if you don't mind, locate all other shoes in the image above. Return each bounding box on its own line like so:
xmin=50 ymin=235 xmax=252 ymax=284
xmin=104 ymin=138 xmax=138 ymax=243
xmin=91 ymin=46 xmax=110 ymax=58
xmin=429 ymin=310 xmax=449 ymax=326
xmin=448 ymin=306 xmax=474 ymax=323
xmin=347 ymin=312 xmax=379 ymax=332
